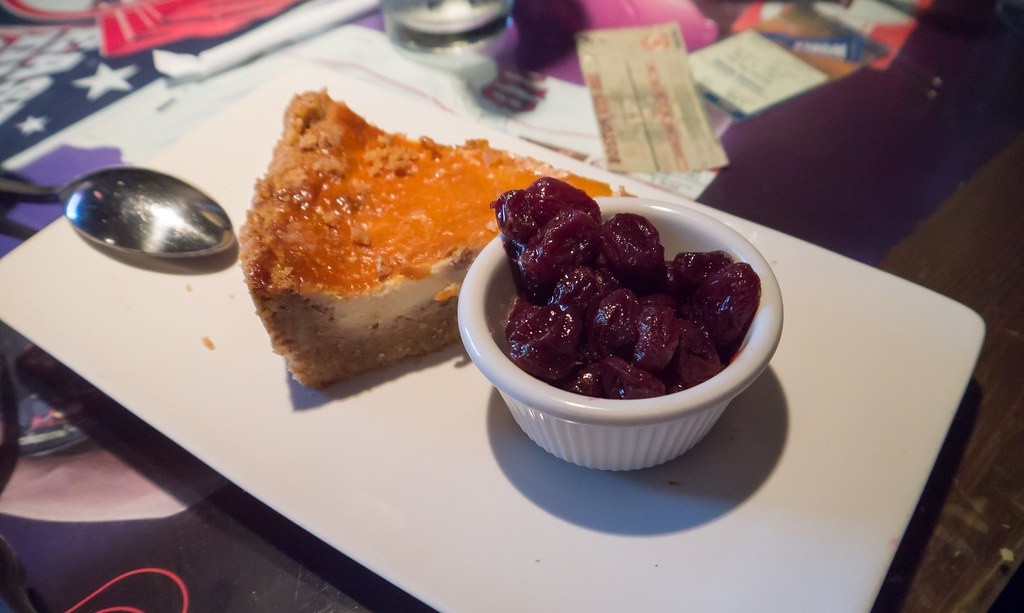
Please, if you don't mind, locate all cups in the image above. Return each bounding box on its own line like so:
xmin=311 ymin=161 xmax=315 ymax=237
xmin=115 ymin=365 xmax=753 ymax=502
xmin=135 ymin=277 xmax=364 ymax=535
xmin=378 ymin=0 xmax=517 ymax=71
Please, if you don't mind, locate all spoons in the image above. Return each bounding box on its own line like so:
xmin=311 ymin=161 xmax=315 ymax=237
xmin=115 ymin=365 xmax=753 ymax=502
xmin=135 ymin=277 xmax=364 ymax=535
xmin=0 ymin=164 xmax=233 ymax=257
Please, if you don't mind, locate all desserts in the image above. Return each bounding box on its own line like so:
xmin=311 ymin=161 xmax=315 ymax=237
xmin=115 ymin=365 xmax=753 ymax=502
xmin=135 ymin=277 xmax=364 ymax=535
xmin=239 ymin=86 xmax=636 ymax=391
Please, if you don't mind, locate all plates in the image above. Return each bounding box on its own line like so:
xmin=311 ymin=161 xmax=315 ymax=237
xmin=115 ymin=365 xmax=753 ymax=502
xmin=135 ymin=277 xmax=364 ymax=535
xmin=0 ymin=66 xmax=987 ymax=613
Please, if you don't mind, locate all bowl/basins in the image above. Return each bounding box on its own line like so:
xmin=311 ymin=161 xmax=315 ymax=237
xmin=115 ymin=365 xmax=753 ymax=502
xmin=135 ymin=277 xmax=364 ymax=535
xmin=457 ymin=197 xmax=781 ymax=469
